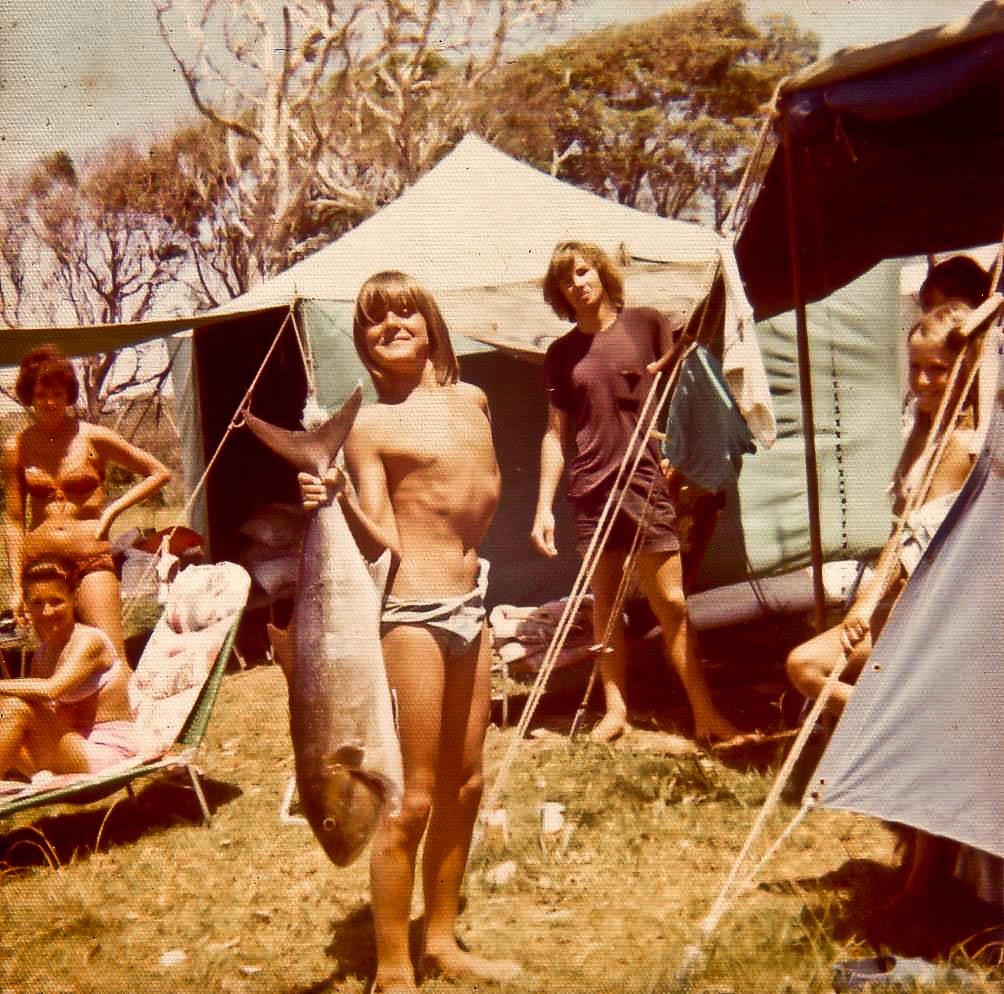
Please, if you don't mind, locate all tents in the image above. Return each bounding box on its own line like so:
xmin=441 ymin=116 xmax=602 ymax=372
xmin=0 ymin=128 xmax=907 ymax=580
xmin=650 ymin=0 xmax=1004 ymax=866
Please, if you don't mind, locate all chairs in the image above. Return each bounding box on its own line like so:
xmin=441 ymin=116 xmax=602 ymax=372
xmin=1 ymin=562 xmax=252 ymax=824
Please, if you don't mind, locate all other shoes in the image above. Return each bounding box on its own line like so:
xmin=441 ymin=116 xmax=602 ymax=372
xmin=832 ymin=953 xmax=937 ymax=992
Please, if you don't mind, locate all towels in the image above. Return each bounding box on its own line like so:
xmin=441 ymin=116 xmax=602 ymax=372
xmin=713 ymin=239 xmax=782 ymax=451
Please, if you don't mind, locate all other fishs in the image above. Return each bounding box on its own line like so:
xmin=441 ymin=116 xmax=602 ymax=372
xmin=237 ymin=380 xmax=402 ymax=869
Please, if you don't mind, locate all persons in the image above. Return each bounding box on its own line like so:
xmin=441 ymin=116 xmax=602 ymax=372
xmin=298 ymin=271 xmax=520 ymax=994
xmin=787 ymin=254 xmax=985 ymax=910
xmin=0 ymin=552 xmax=142 ymax=781
xmin=4 ymin=346 xmax=172 ymax=661
xmin=529 ymin=243 xmax=743 ymax=742
